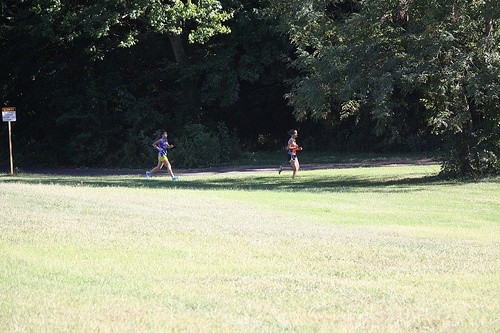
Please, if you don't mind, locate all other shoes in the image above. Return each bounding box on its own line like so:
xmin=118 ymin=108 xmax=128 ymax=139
xmin=146 ymin=171 xmax=150 ymax=178
xmin=278 ymin=165 xmax=283 ymax=174
xmin=172 ymin=176 xmax=181 ymax=181
xmin=293 ymin=177 xmax=299 ymax=180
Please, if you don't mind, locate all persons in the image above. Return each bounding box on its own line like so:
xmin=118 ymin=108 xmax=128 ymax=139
xmin=278 ymin=130 xmax=304 ymax=178
xmin=146 ymin=130 xmax=178 ymax=180
